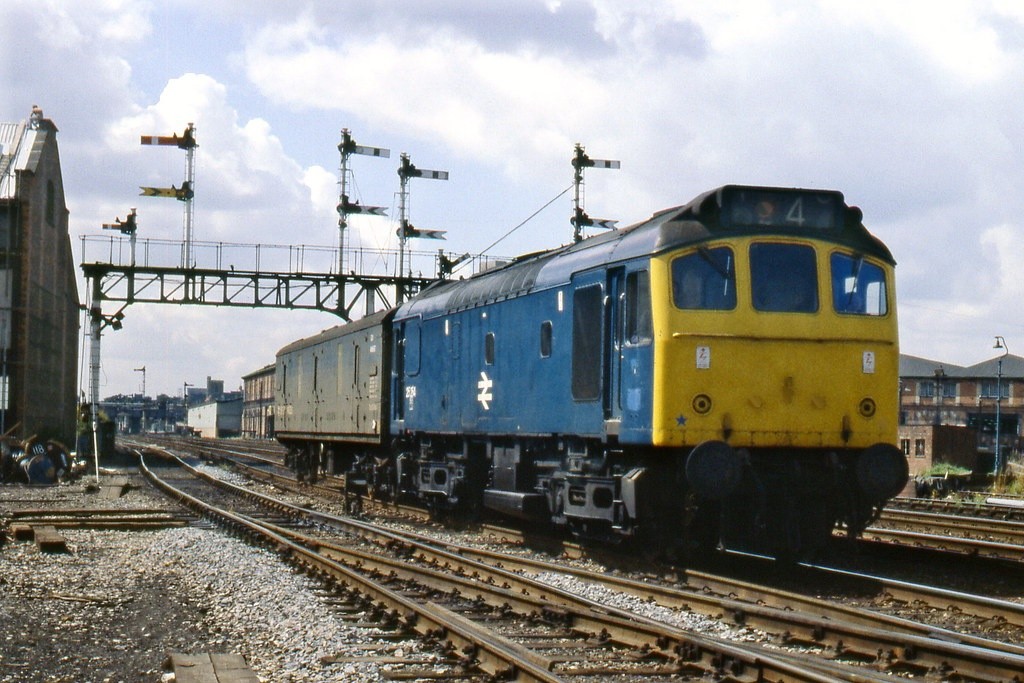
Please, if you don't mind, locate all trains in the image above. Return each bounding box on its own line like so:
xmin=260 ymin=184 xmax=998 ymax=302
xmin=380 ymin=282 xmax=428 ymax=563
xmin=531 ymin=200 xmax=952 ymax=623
xmin=273 ymin=183 xmax=910 ymax=554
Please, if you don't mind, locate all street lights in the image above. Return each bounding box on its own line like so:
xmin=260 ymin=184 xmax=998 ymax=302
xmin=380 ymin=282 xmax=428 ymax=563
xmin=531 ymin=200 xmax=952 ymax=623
xmin=934 ymin=366 xmax=948 ymax=422
xmin=899 ymin=379 xmax=906 ymax=419
xmin=994 ymin=334 xmax=1007 ymax=483
